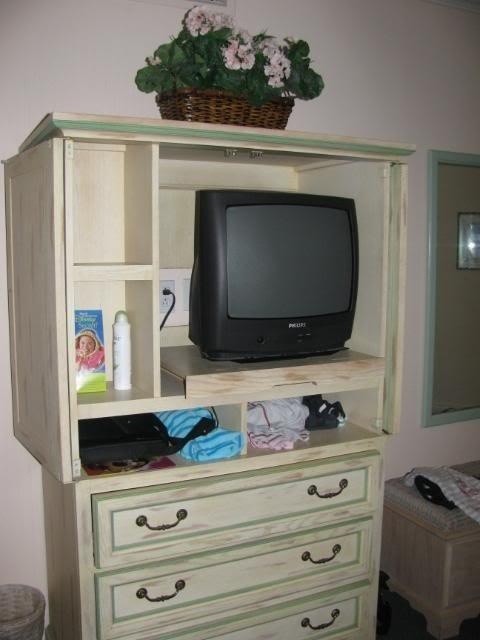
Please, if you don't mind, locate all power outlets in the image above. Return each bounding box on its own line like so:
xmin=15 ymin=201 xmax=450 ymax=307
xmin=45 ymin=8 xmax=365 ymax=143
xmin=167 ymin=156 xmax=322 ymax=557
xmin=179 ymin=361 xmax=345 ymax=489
xmin=158 ymin=279 xmax=175 ymax=314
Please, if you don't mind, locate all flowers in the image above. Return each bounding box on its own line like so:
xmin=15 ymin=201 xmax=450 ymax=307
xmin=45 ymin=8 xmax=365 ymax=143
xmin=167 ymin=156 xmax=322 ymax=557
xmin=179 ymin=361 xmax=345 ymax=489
xmin=133 ymin=4 xmax=325 ymax=106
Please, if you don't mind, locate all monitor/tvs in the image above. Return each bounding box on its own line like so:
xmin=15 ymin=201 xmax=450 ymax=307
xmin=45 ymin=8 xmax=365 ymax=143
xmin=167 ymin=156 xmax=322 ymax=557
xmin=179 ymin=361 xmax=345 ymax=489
xmin=189 ymin=191 xmax=358 ymax=363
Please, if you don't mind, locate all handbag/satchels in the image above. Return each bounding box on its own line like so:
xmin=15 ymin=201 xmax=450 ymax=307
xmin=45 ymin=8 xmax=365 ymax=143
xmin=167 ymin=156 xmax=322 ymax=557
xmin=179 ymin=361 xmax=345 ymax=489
xmin=78 ymin=413 xmax=169 ymax=464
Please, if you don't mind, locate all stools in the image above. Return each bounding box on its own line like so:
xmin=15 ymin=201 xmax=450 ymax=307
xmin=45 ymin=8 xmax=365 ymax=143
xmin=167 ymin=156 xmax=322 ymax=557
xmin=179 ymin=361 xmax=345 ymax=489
xmin=379 ymin=460 xmax=480 ymax=638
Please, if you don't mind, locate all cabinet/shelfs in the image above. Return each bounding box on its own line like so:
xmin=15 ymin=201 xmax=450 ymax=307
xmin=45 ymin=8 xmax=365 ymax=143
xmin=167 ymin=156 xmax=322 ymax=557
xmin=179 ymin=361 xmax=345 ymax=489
xmin=2 ymin=113 xmax=418 ymax=483
xmin=42 ymin=452 xmax=384 ymax=640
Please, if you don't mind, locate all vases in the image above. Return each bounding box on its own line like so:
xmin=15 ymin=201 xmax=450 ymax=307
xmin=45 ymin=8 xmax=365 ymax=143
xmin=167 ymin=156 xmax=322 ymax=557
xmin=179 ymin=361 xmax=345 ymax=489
xmin=155 ymin=87 xmax=293 ymax=129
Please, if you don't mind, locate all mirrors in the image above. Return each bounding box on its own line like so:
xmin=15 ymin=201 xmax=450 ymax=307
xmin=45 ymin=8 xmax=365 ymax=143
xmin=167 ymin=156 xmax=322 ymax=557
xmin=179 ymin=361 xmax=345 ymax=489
xmin=420 ymin=148 xmax=480 ymax=427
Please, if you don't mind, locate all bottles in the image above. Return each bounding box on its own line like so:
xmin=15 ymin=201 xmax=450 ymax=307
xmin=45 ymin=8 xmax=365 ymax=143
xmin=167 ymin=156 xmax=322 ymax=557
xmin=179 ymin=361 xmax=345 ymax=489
xmin=111 ymin=310 xmax=135 ymax=393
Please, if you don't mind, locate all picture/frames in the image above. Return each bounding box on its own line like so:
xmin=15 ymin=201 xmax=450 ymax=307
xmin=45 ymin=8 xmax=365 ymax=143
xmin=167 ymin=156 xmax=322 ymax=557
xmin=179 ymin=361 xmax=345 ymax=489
xmin=455 ymin=212 xmax=480 ymax=270
